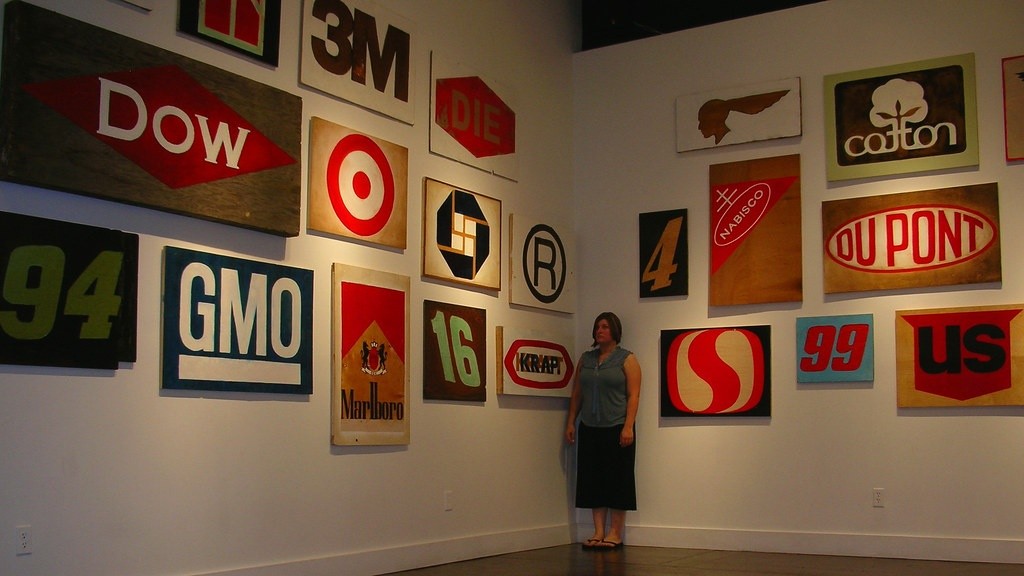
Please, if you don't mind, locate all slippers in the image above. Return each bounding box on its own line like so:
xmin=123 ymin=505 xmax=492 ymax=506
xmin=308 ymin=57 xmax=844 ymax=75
xmin=594 ymin=539 xmax=623 ymax=551
xmin=582 ymin=539 xmax=603 ymax=550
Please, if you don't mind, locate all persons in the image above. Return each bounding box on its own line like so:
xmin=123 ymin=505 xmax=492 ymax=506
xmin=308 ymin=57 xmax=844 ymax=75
xmin=565 ymin=311 xmax=641 ymax=551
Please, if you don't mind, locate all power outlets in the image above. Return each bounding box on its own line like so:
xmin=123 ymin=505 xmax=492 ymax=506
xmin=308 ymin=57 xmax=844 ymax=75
xmin=873 ymin=488 xmax=885 ymax=507
xmin=15 ymin=525 xmax=33 ymax=556
xmin=444 ymin=490 xmax=453 ymax=512
